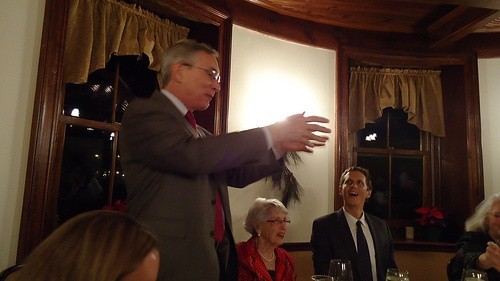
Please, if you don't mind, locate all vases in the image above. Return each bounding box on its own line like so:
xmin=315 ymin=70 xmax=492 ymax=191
xmin=428 ymin=225 xmax=442 ymax=240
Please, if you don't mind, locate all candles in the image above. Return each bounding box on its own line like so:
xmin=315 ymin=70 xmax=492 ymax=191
xmin=405 ymin=225 xmax=415 ymax=240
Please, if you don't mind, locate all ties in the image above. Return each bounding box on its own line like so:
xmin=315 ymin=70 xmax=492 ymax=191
xmin=356 ymin=221 xmax=373 ymax=281
xmin=186 ymin=111 xmax=225 ymax=243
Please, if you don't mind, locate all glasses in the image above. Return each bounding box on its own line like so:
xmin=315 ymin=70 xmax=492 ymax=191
xmin=181 ymin=63 xmax=221 ymax=83
xmin=266 ymin=218 xmax=291 ymax=225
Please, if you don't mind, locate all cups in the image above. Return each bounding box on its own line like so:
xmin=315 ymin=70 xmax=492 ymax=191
xmin=385 ymin=268 xmax=409 ymax=281
xmin=310 ymin=274 xmax=334 ymax=281
xmin=328 ymin=259 xmax=353 ymax=281
xmin=465 ymin=269 xmax=488 ymax=281
xmin=406 ymin=226 xmax=414 ymax=239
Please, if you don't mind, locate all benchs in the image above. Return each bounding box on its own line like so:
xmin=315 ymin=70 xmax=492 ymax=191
xmin=280 ymin=238 xmax=454 ymax=281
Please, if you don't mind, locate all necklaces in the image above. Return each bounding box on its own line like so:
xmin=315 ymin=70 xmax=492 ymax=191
xmin=257 ymin=248 xmax=275 ymax=262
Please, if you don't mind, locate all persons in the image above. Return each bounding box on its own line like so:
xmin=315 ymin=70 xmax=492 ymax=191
xmin=12 ymin=210 xmax=161 ymax=281
xmin=118 ymin=41 xmax=332 ymax=281
xmin=452 ymin=194 xmax=500 ymax=281
xmin=235 ymin=198 xmax=299 ymax=281
xmin=309 ymin=167 xmax=399 ymax=281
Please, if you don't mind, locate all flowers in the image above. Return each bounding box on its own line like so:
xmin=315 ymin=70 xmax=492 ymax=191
xmin=414 ymin=203 xmax=449 ymax=228
